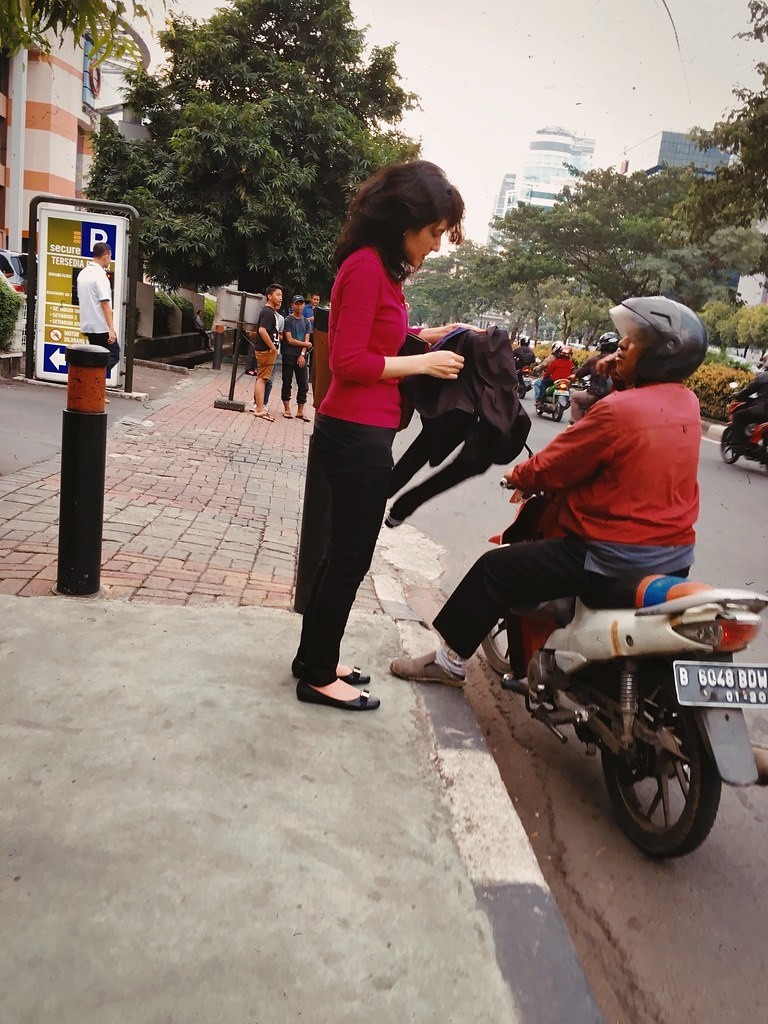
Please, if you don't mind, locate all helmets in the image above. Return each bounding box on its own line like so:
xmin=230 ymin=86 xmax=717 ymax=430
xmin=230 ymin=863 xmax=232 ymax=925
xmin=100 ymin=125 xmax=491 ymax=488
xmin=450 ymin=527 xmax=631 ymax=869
xmin=621 ymin=295 xmax=708 ymax=381
xmin=559 ymin=345 xmax=572 ymax=358
xmin=520 ymin=336 xmax=530 ymax=346
xmin=762 ymin=351 xmax=768 ymax=366
xmin=599 ymin=332 xmax=620 ymax=351
xmin=552 ymin=341 xmax=564 ymax=354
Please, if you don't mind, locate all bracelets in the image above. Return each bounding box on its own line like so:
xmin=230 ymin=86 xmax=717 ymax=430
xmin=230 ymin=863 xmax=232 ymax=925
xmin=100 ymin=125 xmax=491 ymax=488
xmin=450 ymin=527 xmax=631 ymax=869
xmin=301 ymin=351 xmax=305 ymax=357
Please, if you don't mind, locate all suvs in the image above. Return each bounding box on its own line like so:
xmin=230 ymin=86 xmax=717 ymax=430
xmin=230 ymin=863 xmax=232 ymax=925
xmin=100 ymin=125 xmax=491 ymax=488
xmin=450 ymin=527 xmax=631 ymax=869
xmin=0 ymin=247 xmax=38 ymax=295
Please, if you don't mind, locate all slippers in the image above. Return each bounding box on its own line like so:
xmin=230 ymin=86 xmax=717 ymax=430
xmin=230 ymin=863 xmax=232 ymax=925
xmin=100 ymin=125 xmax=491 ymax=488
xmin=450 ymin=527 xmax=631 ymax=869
xmin=295 ymin=414 xmax=311 ymax=422
xmin=254 ymin=411 xmax=275 ymax=422
xmin=281 ymin=411 xmax=293 ymax=419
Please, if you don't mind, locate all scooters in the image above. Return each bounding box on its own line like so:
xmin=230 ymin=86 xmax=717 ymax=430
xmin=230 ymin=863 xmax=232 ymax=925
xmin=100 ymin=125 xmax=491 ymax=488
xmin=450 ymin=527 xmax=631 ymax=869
xmin=533 ymin=369 xmax=571 ymax=421
xmin=516 ymin=366 xmax=533 ymax=400
xmin=717 ymin=382 xmax=768 ymax=471
xmin=483 ymin=440 xmax=768 ymax=860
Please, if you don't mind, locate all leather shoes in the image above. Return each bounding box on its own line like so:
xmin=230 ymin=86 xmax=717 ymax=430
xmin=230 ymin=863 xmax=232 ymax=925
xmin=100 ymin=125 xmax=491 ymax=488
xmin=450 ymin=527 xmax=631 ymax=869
xmin=291 ymin=658 xmax=371 ymax=685
xmin=297 ymin=680 xmax=380 ymax=710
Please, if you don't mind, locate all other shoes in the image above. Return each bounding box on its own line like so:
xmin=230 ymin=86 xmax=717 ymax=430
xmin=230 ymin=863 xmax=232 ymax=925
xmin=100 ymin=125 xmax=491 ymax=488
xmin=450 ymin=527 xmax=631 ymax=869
xmin=533 ymin=401 xmax=542 ymax=406
xmin=389 ymin=650 xmax=468 ymax=688
xmin=245 ymin=369 xmax=258 ymax=376
xmin=537 ymin=403 xmax=544 ymax=409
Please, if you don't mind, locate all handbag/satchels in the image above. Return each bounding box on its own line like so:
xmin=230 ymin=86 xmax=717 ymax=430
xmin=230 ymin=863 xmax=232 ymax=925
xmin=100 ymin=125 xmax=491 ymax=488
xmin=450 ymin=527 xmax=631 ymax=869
xmin=311 ymin=305 xmax=431 ymax=430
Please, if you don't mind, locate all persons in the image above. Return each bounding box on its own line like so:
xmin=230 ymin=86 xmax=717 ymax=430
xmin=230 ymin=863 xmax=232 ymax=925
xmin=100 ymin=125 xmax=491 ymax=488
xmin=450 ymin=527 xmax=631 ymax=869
xmin=194 ymin=309 xmax=209 ymax=352
xmin=77 ymin=243 xmax=121 ymax=402
xmin=389 ymin=297 xmax=707 ymax=688
xmin=288 ymin=161 xmax=489 ymax=710
xmin=252 ymin=284 xmax=323 ymax=423
xmin=729 ymin=353 xmax=768 ymax=451
xmin=514 ymin=332 xmax=622 ymax=428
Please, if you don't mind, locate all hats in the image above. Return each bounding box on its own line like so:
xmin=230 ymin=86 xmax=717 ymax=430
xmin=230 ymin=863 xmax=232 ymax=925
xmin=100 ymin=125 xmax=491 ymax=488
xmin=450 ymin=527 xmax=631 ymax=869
xmin=292 ymin=295 xmax=305 ymax=303
